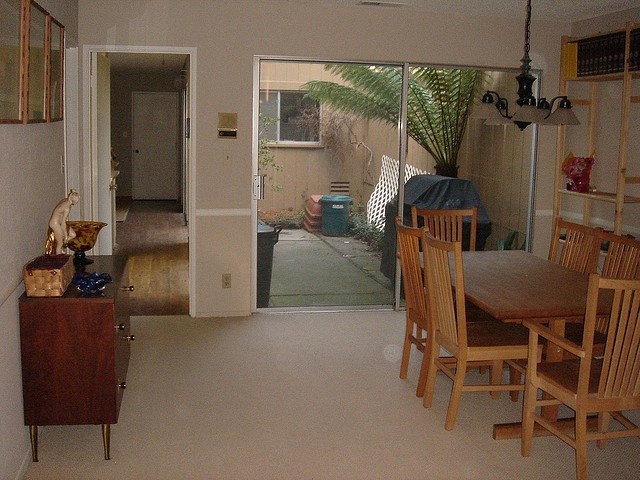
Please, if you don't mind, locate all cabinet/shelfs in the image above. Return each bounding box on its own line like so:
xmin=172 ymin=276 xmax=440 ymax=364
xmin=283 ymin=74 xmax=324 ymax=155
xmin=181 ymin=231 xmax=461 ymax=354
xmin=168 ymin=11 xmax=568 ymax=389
xmin=18 ymin=255 xmax=135 ymax=460
xmin=553 ymin=23 xmax=640 ymax=236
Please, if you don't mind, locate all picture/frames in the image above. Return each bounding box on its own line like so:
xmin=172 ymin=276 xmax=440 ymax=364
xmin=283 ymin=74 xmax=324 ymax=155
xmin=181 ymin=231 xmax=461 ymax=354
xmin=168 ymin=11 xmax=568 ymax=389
xmin=24 ymin=0 xmax=48 ymax=123
xmin=48 ymin=17 xmax=65 ymax=123
xmin=0 ymin=1 xmax=23 ymax=124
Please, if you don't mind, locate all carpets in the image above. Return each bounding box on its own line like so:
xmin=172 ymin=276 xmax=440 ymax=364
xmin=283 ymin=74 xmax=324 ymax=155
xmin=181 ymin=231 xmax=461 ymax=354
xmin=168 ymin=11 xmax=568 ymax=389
xmin=116 ymin=199 xmax=132 ymax=223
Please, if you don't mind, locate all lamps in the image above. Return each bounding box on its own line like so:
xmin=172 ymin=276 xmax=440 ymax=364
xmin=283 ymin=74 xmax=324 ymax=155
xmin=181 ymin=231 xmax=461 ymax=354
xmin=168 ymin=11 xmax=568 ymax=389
xmin=469 ymin=1 xmax=580 ymax=131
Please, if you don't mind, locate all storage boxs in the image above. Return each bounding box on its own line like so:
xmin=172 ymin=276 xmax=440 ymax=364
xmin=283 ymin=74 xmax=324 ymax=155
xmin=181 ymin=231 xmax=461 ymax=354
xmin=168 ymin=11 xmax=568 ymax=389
xmin=23 ymin=253 xmax=76 ymax=297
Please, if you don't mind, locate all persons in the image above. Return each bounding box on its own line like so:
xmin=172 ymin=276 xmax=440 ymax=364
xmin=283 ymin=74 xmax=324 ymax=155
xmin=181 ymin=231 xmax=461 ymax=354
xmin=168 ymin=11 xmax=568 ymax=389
xmin=48 ymin=190 xmax=80 ymax=255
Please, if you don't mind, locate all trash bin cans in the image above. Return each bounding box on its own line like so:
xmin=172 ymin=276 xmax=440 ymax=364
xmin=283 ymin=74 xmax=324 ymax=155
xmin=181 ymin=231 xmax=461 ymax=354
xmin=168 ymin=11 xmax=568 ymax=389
xmin=318 ymin=195 xmax=353 ymax=237
xmin=258 ymin=225 xmax=282 ymax=308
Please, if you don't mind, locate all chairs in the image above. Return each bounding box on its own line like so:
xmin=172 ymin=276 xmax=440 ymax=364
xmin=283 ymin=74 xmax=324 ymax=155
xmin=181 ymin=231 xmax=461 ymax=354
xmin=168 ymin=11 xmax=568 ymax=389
xmin=410 ymin=207 xmax=494 ymax=349
xmin=548 ymin=217 xmax=603 ymax=275
xmin=521 ymin=276 xmax=639 ymax=480
xmin=394 ymin=214 xmax=497 ymax=398
xmin=510 ymin=228 xmax=640 ymax=403
xmin=424 ymin=232 xmax=545 ymax=431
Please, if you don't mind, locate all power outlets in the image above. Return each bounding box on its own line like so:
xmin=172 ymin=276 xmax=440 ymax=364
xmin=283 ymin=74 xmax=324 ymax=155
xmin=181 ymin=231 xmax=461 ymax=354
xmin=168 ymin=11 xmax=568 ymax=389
xmin=223 ymin=274 xmax=232 ymax=288
